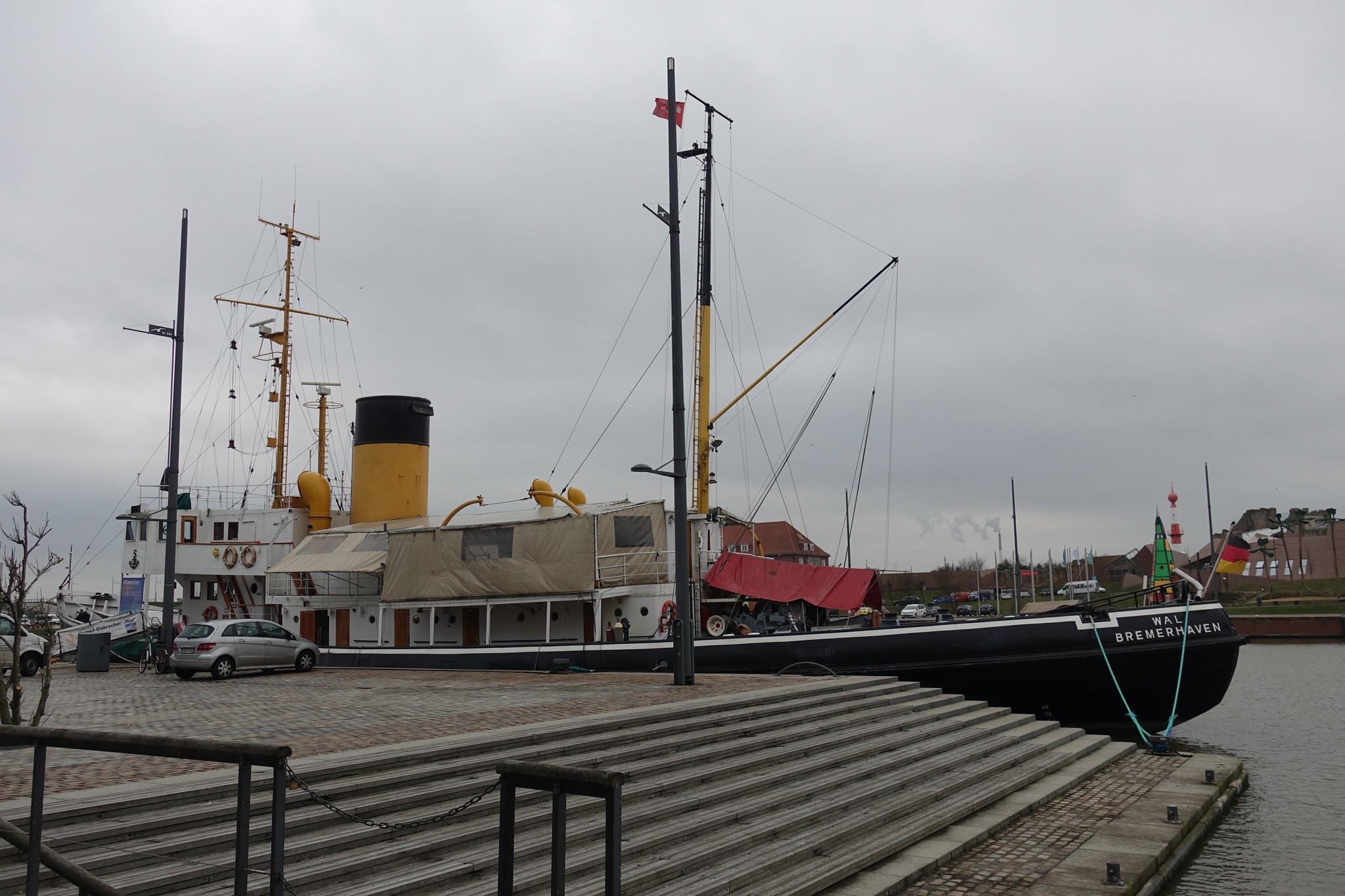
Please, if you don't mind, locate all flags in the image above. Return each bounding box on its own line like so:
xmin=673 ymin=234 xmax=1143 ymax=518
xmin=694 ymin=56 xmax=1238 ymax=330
xmin=1215 ymin=532 xmax=1252 ymax=576
xmin=1073 ymin=548 xmax=1081 ymax=565
xmin=652 ymin=98 xmax=685 ymax=129
xmin=1087 ymin=549 xmax=1093 ymax=565
xmin=1062 ymin=549 xmax=1067 ymax=565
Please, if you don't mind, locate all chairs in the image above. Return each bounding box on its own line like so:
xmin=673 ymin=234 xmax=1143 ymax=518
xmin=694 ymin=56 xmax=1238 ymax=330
xmin=239 ymin=626 xmax=248 ymax=637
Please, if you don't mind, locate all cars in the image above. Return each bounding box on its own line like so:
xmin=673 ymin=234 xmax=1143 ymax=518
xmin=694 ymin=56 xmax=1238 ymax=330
xmin=1040 ymin=588 xmax=1050 ymax=597
xmin=169 ymin=618 xmax=321 ymax=681
xmin=925 ymin=602 xmax=941 ymax=617
xmin=1053 ymin=589 xmax=1058 ymax=596
xmin=1099 ymin=585 xmax=1107 ymax=593
xmin=900 ymin=604 xmax=927 ymax=618
xmin=1020 ymin=591 xmax=1031 ymax=598
xmin=933 ymin=595 xmax=956 ymax=604
xmin=828 ymin=608 xmax=840 ymax=618
xmin=854 ymin=598 xmax=890 ymax=615
xmin=978 ymin=604 xmax=997 ymax=616
xmin=956 ymin=605 xmax=977 ymax=617
xmin=20 ymin=614 xmax=34 ymax=628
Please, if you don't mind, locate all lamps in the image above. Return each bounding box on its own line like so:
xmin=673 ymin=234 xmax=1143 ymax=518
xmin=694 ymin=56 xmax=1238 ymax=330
xmin=709 ymin=473 xmax=717 ymax=482
xmin=229 ymin=389 xmax=236 ymax=399
xmin=284 ymin=224 xmax=289 ymax=227
xmin=714 ymin=508 xmax=720 ymax=519
xmin=482 ymin=611 xmax=486 ymax=615
xmin=254 ymin=576 xmax=259 ymax=582
xmin=657 ymin=548 xmax=662 ymax=556
xmin=292 ymin=235 xmax=302 ymax=247
xmin=328 ymin=608 xmax=332 ymax=616
xmin=358 ymin=606 xmax=364 ymax=615
xmin=709 ymin=434 xmax=714 ymax=438
xmin=281 ymin=607 xmax=288 ymax=615
xmin=710 ymin=440 xmax=723 ymax=453
xmin=250 ymin=318 xmax=275 ymax=327
xmin=303 ymin=601 xmax=308 ymax=607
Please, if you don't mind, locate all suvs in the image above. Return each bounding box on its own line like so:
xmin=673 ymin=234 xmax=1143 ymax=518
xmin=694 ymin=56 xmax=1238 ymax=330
xmin=893 ymin=595 xmax=921 ymax=606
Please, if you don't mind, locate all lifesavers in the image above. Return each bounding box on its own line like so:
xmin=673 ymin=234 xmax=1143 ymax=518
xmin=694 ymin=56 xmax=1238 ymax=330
xmin=222 ymin=546 xmax=238 ymax=568
xmin=663 ymin=600 xmax=676 ymax=626
xmin=204 ymin=606 xmax=218 ymax=620
xmin=241 ymin=546 xmax=256 ymax=567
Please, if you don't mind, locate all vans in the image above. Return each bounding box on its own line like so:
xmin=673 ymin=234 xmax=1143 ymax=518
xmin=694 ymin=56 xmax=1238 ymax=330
xmin=35 ymin=614 xmax=60 ymax=626
xmin=950 ymin=590 xmax=996 ymax=603
xmin=1057 ymin=580 xmax=1100 ymax=595
xmin=999 ymin=589 xmax=1013 ymax=600
xmin=0 ymin=613 xmax=50 ymax=677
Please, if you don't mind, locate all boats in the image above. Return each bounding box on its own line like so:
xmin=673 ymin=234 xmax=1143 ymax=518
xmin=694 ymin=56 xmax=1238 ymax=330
xmin=54 ymin=57 xmax=1254 ymax=744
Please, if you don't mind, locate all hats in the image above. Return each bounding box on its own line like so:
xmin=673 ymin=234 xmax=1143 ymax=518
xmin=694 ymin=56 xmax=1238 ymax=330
xmin=621 ymin=618 xmax=630 ymax=624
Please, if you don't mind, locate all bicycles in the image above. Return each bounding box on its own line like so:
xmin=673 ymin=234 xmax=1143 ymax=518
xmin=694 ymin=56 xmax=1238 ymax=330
xmin=139 ymin=633 xmax=169 ymax=675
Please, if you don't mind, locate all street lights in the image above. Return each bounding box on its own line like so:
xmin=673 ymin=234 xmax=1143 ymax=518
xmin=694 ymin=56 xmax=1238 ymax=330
xmin=630 ymin=463 xmax=695 ymax=686
xmin=1208 ymin=566 xmax=1219 ymax=600
xmin=1011 ymin=572 xmax=1022 ymax=615
xmin=115 ymin=514 xmax=177 ymax=673
